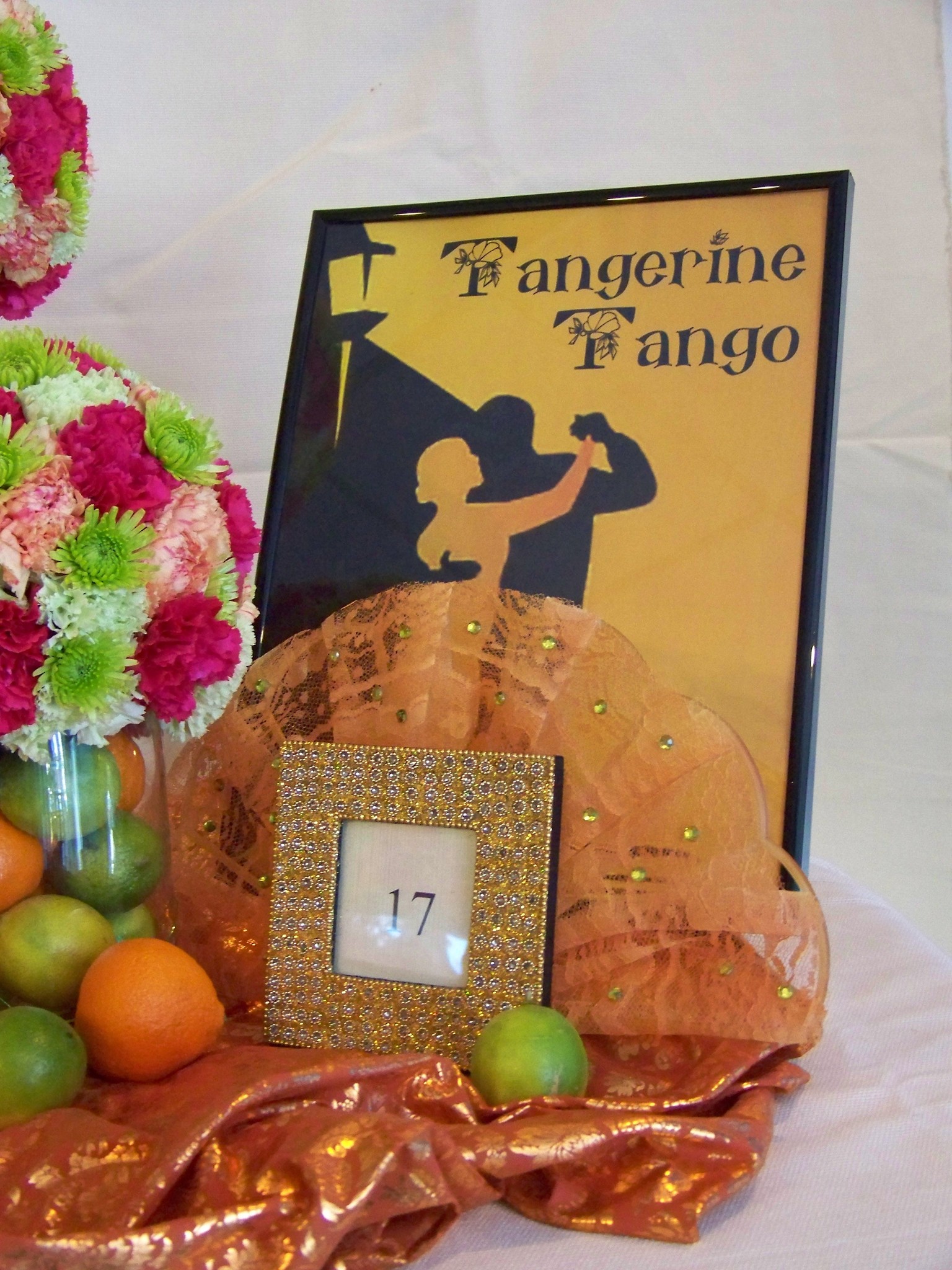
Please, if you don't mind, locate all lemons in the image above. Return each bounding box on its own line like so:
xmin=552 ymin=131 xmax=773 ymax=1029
xmin=0 ymin=737 xmax=592 ymax=1123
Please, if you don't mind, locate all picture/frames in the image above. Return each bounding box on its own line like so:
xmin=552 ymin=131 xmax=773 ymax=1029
xmin=240 ymin=164 xmax=857 ymax=894
xmin=266 ymin=741 xmax=563 ymax=1064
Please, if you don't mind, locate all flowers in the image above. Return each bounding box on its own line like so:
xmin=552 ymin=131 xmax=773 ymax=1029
xmin=0 ymin=1 xmax=269 ymax=763
